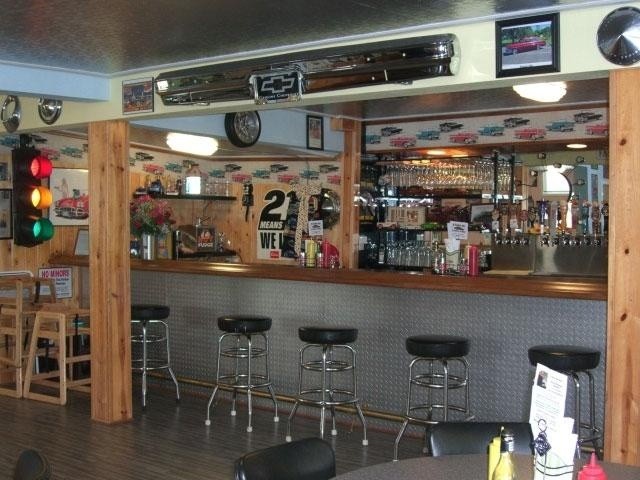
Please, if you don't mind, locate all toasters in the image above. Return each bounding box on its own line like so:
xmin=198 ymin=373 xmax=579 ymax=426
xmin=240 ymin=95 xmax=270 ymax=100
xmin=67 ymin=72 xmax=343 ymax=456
xmin=386 ymin=163 xmax=516 ymax=191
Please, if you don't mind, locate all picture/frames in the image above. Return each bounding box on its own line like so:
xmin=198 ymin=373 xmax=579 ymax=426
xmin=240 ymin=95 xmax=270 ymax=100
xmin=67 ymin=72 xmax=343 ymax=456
xmin=0 ymin=161 xmax=14 ymax=240
xmin=306 ymin=115 xmax=325 ymax=151
xmin=495 ymin=11 xmax=561 ymax=78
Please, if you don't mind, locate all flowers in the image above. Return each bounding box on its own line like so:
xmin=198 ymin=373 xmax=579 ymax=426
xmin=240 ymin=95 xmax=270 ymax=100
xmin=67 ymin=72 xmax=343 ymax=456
xmin=128 ymin=193 xmax=176 ymax=240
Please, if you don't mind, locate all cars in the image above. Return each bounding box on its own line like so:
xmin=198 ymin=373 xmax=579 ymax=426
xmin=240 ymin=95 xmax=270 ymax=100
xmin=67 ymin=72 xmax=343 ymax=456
xmin=55 ymin=189 xmax=91 ymax=222
xmin=361 ymin=108 xmax=609 ymax=145
xmin=505 ymin=36 xmax=546 ymax=55
xmin=1 ymin=131 xmax=89 ymax=162
xmin=129 ymin=151 xmax=343 ymax=185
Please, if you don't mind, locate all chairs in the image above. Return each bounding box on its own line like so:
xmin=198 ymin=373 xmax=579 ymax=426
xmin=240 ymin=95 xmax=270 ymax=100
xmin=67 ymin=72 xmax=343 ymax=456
xmin=425 ymin=423 xmax=535 ymax=456
xmin=234 ymin=437 xmax=336 ymax=480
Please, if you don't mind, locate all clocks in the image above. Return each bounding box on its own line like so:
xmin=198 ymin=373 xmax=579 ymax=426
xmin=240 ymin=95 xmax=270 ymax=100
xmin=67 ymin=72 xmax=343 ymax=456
xmin=224 ymin=110 xmax=263 ymax=149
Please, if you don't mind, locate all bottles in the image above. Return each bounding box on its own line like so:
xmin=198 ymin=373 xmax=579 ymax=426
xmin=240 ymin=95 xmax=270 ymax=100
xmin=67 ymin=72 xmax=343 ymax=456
xmin=379 ymin=230 xmax=479 ymax=276
xmin=491 ymin=428 xmax=520 ymax=480
xmin=299 ymin=236 xmax=332 ymax=269
xmin=144 ymin=172 xmax=235 ymax=197
xmin=578 ymin=453 xmax=607 ymax=480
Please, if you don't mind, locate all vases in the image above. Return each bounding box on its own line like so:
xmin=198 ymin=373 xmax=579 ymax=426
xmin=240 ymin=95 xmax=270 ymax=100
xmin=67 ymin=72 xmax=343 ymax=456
xmin=140 ymin=230 xmax=159 ymax=261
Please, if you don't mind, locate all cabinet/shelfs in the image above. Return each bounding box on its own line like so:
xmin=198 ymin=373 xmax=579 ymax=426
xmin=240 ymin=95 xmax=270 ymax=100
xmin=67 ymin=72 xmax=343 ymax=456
xmin=135 ymin=192 xmax=237 ymax=258
xmin=375 ymin=151 xmax=517 ymax=246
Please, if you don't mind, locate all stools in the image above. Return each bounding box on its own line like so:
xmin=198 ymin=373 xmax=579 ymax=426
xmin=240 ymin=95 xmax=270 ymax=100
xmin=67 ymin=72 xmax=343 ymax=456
xmin=22 ymin=308 xmax=90 ymax=406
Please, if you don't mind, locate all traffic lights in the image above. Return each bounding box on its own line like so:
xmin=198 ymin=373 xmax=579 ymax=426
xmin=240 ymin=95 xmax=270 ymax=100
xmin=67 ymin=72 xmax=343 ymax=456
xmin=10 ymin=133 xmax=55 ymax=248
